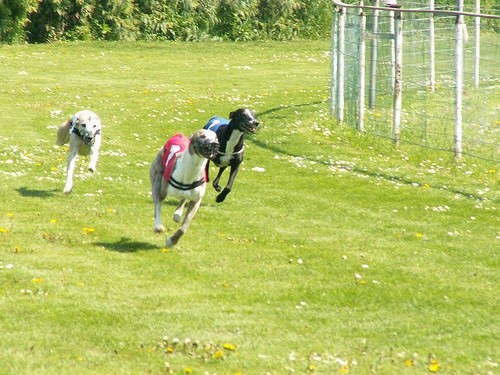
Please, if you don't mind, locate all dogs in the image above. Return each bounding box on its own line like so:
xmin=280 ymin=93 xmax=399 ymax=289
xmin=203 ymin=108 xmax=261 ymax=203
xmin=150 ymin=129 xmax=220 ymax=247
xmin=56 ymin=109 xmax=102 ymax=193
